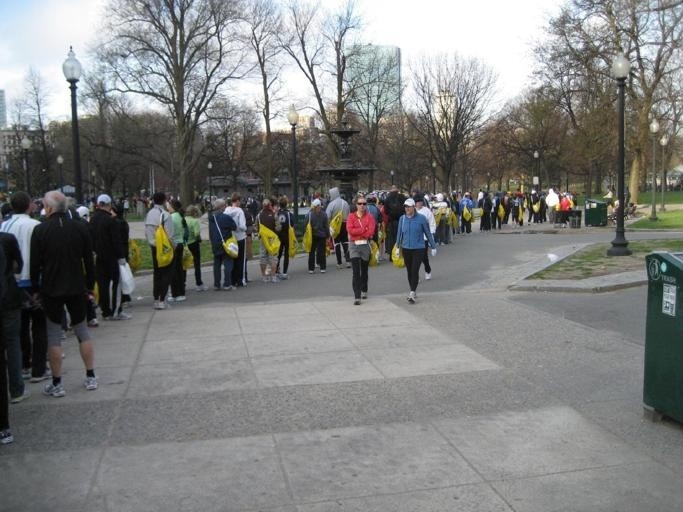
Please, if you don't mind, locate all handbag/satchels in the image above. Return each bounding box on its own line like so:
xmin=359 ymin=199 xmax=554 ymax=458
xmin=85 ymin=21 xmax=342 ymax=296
xmin=391 ymin=241 xmax=405 ymax=268
xmin=118 ymin=262 xmax=136 ymax=295
xmin=155 ymin=211 xmax=343 ymax=267
xmin=369 ymin=240 xmax=381 ymax=267
xmin=127 ymin=239 xmax=141 ymax=273
xmin=433 ymin=199 xmax=575 ymax=226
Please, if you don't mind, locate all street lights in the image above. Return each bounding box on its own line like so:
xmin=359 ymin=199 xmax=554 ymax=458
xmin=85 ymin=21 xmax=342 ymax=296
xmin=647 ymin=119 xmax=658 ymax=221
xmin=391 ymin=170 xmax=395 ymax=186
xmin=533 ymin=150 xmax=540 ymax=193
xmin=91 ymin=170 xmax=96 ymax=201
xmin=205 ymin=159 xmax=214 ymax=205
xmin=61 ymin=43 xmax=83 ymax=202
xmin=487 ymin=172 xmax=491 ymax=188
xmin=57 ymin=153 xmax=64 ymax=195
xmin=455 ymin=172 xmax=459 ymax=197
xmin=285 ymin=100 xmax=300 ymax=224
xmin=607 ymin=47 xmax=631 ymax=258
xmin=432 ymin=161 xmax=437 ymax=196
xmin=19 ymin=134 xmax=31 ymax=198
xmin=658 ymin=135 xmax=667 ymax=212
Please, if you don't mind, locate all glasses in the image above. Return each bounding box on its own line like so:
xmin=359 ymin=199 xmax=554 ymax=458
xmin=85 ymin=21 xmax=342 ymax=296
xmin=358 ymin=203 xmax=367 ymax=205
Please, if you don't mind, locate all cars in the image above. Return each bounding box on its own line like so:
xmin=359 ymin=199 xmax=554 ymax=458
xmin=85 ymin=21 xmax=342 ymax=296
xmin=634 ymin=169 xmax=682 ymax=192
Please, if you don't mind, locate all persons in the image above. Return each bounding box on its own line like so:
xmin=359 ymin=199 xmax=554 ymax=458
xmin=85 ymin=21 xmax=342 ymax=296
xmin=2 ymin=185 xmax=133 ymax=443
xmin=602 ymin=185 xmax=615 ymax=207
xmin=478 ymin=187 xmax=578 ymax=233
xmin=397 ymin=197 xmax=437 ymax=303
xmin=139 ymin=191 xmax=296 ymax=309
xmin=346 ymin=197 xmax=376 ymax=305
xmin=304 ymin=186 xmax=475 ymax=274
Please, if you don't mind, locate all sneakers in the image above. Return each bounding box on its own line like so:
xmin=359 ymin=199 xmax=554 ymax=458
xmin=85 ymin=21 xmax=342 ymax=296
xmin=362 ymin=293 xmax=368 ymax=299
xmin=2 ymin=301 xmax=132 ymax=443
xmin=153 ymin=262 xmax=352 ymax=309
xmin=425 ymin=272 xmax=431 ymax=280
xmin=408 ymin=291 xmax=416 ymax=302
xmin=354 ymin=299 xmax=361 ymax=305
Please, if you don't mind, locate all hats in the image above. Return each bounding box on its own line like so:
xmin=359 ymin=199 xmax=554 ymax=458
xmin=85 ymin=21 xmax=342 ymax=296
xmin=97 ymin=193 xmax=111 ymax=206
xmin=76 ymin=205 xmax=89 ymax=220
xmin=403 ymin=198 xmax=415 ymax=206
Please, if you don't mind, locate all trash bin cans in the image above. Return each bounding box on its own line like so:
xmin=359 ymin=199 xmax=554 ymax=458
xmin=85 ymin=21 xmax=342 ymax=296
xmin=585 ymin=199 xmax=607 ymax=227
xmin=643 ymin=251 xmax=683 ymax=423
xmin=569 ymin=210 xmax=581 ymax=229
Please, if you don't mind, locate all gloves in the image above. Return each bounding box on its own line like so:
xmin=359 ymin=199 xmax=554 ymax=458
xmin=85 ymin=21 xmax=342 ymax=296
xmin=432 ymin=248 xmax=437 ymax=256
